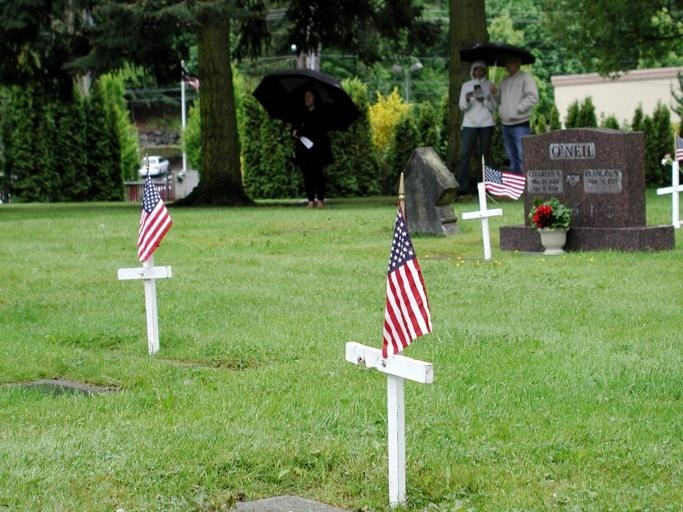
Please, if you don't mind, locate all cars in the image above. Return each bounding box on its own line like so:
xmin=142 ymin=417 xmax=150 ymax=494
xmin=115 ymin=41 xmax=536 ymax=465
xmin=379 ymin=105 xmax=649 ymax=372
xmin=138 ymin=154 xmax=170 ymax=178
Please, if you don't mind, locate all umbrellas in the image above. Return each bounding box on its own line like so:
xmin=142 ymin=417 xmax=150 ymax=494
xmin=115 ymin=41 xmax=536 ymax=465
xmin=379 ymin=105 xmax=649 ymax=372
xmin=249 ymin=63 xmax=364 ymax=145
xmin=450 ymin=31 xmax=544 ymax=69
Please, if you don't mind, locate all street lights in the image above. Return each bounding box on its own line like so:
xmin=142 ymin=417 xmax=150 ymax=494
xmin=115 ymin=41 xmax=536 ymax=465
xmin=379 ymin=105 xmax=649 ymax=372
xmin=389 ymin=61 xmax=424 ymax=107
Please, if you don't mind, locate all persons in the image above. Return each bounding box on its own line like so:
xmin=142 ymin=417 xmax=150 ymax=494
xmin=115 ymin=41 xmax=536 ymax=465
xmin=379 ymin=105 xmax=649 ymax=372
xmin=489 ymin=61 xmax=540 ymax=178
xmin=450 ymin=62 xmax=499 ymax=193
xmin=288 ymin=90 xmax=339 ymax=209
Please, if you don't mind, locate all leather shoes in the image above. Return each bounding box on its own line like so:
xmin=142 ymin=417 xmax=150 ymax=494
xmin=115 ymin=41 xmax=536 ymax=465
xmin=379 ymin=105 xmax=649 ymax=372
xmin=305 ymin=200 xmax=324 ymax=208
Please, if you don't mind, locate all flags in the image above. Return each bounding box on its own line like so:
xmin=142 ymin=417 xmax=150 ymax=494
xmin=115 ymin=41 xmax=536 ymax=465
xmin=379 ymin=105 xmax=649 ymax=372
xmin=376 ymin=216 xmax=430 ymax=364
xmin=181 ymin=65 xmax=200 ymax=91
xmin=477 ymin=152 xmax=525 ymax=203
xmin=134 ymin=165 xmax=177 ymax=270
xmin=675 ymin=135 xmax=683 ymax=162
